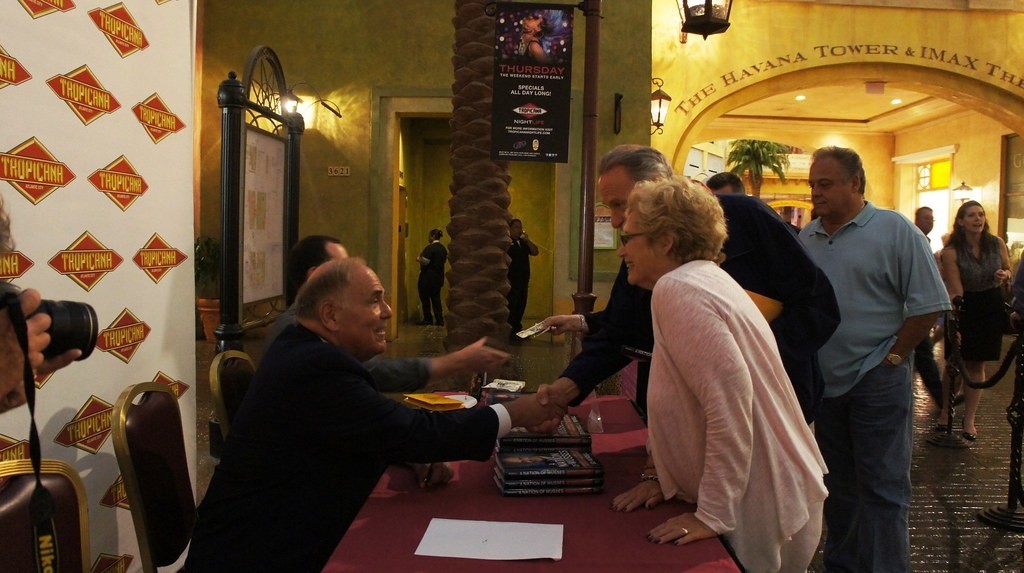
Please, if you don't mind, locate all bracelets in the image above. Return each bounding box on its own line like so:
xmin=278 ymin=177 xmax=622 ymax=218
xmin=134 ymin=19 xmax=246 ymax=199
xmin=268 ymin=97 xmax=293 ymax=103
xmin=642 ymin=475 xmax=658 ymax=481
xmin=578 ymin=315 xmax=585 ymax=330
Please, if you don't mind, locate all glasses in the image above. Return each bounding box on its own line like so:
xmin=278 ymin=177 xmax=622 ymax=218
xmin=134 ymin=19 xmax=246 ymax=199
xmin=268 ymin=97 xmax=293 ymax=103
xmin=620 ymin=232 xmax=649 ymax=247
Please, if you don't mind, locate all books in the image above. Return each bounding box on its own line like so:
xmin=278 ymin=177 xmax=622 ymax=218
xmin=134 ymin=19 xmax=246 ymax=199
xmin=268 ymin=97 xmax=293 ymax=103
xmin=479 ymin=392 xmax=604 ymax=494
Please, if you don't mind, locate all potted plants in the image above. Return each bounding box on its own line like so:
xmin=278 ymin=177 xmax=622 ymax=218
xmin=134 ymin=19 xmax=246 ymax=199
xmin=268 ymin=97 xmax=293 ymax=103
xmin=194 ymin=237 xmax=223 ymax=344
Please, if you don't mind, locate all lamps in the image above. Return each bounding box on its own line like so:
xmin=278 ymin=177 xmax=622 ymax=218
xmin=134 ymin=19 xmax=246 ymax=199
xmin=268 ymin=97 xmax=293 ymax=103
xmin=675 ymin=0 xmax=734 ymax=40
xmin=276 ymin=82 xmax=343 ymax=135
xmin=650 ymin=78 xmax=672 ymax=136
xmin=951 ymin=181 xmax=974 ymax=204
xmin=863 ymin=79 xmax=886 ymax=96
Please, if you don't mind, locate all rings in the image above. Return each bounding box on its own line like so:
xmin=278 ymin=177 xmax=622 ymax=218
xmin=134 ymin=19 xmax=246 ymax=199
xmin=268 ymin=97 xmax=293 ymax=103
xmin=682 ymin=528 xmax=688 ymax=533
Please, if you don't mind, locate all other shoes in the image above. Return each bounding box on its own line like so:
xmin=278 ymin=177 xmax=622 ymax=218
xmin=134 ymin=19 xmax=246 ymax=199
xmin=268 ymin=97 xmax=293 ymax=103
xmin=435 ymin=321 xmax=445 ymax=327
xmin=941 ymin=392 xmax=965 ymax=409
xmin=938 ymin=423 xmax=947 ymax=431
xmin=509 ymin=332 xmax=533 ymax=345
xmin=962 ymin=418 xmax=977 ymax=442
xmin=416 ymin=319 xmax=434 ymax=326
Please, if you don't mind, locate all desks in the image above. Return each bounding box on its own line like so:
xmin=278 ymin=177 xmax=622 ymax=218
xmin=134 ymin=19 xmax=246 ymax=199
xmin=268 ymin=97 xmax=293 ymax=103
xmin=323 ymin=392 xmax=747 ymax=573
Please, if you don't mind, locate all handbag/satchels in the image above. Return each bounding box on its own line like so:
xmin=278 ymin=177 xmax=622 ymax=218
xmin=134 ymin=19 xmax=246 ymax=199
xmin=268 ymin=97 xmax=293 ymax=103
xmin=1003 ymin=275 xmax=1020 ymax=335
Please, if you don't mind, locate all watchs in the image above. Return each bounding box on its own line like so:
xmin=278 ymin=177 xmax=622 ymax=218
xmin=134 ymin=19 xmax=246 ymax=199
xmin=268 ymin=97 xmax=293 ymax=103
xmin=887 ymin=353 xmax=902 ymax=365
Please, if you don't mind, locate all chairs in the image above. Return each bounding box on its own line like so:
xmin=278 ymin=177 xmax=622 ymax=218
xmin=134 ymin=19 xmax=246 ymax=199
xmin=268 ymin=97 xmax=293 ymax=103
xmin=0 ymin=460 xmax=92 ymax=573
xmin=111 ymin=382 xmax=197 ymax=573
xmin=209 ymin=351 xmax=257 ymax=443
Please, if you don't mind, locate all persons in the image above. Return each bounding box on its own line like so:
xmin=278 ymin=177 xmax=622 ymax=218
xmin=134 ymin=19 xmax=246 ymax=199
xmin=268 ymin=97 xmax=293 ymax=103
xmin=611 ymin=179 xmax=830 ymax=573
xmin=503 ymin=453 xmax=558 ymax=466
xmin=528 ymin=144 xmax=842 ymax=435
xmin=707 ymin=172 xmax=745 ymax=194
xmin=0 ymin=197 xmax=82 ymax=414
xmin=914 ymin=201 xmax=1024 ymax=440
xmin=519 ymin=16 xmax=553 ymax=61
xmin=260 ymin=235 xmax=512 ymax=391
xmin=416 ymin=229 xmax=447 ymax=326
xmin=181 ymin=258 xmax=567 ymax=573
xmin=508 ymin=219 xmax=539 ymax=340
xmin=798 ymin=148 xmax=952 ymax=573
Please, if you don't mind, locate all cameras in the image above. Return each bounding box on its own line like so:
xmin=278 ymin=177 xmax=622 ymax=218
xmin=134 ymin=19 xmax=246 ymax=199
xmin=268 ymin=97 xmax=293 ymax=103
xmin=0 ymin=280 xmax=99 ymax=361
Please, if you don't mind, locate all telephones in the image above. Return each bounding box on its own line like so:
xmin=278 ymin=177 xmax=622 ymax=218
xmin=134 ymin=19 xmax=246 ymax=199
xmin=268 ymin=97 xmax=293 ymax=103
xmin=520 ymin=230 xmax=526 ymax=238
